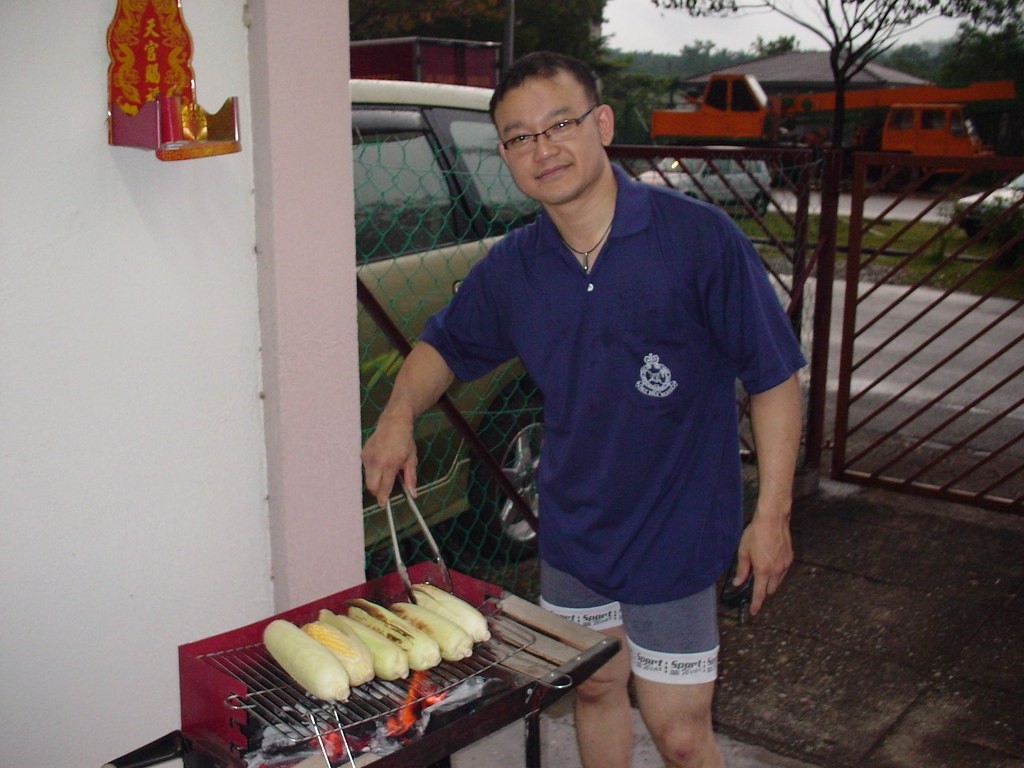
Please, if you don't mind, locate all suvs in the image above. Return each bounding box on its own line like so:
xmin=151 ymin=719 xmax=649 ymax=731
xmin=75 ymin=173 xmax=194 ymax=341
xmin=633 ymin=147 xmax=773 ymax=217
xmin=349 ymin=77 xmax=570 ymax=583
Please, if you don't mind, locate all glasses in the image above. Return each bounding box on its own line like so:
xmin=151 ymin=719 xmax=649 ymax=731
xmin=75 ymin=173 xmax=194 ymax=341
xmin=501 ymin=104 xmax=595 ymax=154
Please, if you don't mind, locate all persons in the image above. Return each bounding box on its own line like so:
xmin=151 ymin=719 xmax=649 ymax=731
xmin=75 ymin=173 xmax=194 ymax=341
xmin=356 ymin=52 xmax=805 ymax=768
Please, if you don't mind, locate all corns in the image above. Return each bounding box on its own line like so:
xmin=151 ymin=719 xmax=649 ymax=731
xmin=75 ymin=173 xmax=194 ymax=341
xmin=264 ymin=582 xmax=491 ymax=698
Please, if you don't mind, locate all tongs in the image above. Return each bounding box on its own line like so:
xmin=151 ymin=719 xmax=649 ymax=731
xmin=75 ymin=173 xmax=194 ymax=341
xmin=385 ymin=472 xmax=454 ymax=607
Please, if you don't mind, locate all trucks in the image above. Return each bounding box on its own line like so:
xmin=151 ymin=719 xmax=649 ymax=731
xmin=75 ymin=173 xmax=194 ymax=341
xmin=347 ymin=36 xmax=500 ymax=92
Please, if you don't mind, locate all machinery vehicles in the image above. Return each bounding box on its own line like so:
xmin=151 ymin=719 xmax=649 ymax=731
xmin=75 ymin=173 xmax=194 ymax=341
xmin=646 ymin=60 xmax=1022 ymax=199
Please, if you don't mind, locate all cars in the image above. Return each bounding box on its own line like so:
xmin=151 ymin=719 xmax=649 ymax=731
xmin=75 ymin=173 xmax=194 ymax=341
xmin=950 ymin=170 xmax=1024 ymax=247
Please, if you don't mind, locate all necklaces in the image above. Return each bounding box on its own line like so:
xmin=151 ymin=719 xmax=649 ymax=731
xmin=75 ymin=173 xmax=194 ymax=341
xmin=557 ymin=216 xmax=617 ymax=271
